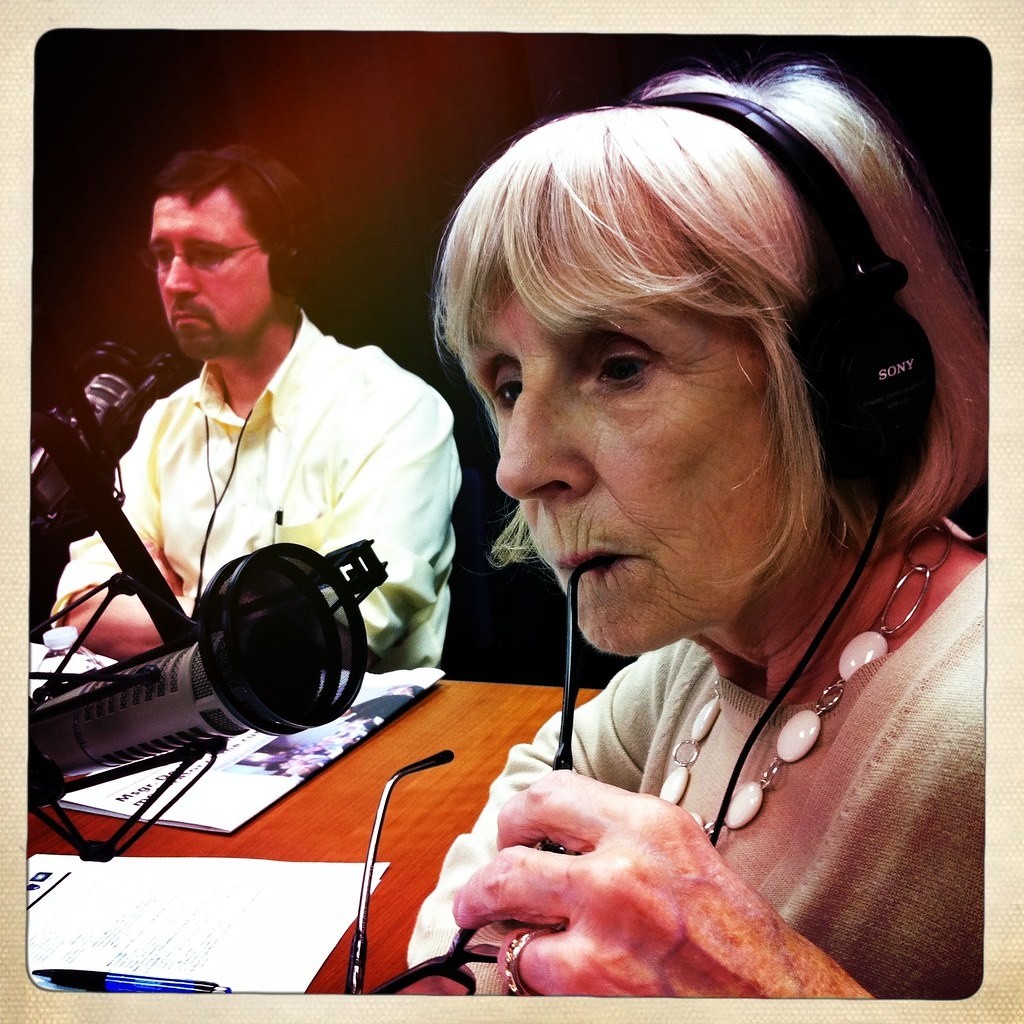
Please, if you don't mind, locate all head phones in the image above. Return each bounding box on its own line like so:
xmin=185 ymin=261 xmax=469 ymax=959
xmin=187 ymin=152 xmax=320 ymax=298
xmin=636 ymin=92 xmax=934 ymax=501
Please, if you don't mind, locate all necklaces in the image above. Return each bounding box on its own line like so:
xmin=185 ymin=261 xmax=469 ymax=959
xmin=659 ymin=521 xmax=955 ymax=841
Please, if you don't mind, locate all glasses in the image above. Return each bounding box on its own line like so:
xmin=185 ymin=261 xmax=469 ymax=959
xmin=139 ymin=241 xmax=261 ymax=271
xmin=344 ymin=554 xmax=620 ymax=996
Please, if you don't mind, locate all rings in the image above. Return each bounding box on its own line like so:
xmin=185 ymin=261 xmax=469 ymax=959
xmin=501 ymin=931 xmax=559 ymax=996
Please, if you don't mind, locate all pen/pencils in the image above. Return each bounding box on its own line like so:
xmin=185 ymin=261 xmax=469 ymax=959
xmin=32 ymin=969 xmax=232 ymax=993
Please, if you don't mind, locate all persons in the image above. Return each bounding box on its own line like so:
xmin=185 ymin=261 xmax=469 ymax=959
xmin=48 ymin=147 xmax=462 ymax=676
xmin=405 ymin=74 xmax=984 ymax=999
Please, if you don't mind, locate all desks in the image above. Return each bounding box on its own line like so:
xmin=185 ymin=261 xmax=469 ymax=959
xmin=25 ymin=671 xmax=607 ymax=994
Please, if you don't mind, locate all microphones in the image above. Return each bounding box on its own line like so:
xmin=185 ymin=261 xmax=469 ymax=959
xmin=28 ymin=612 xmax=326 ymax=776
xmin=30 ymin=372 xmax=138 ymax=528
